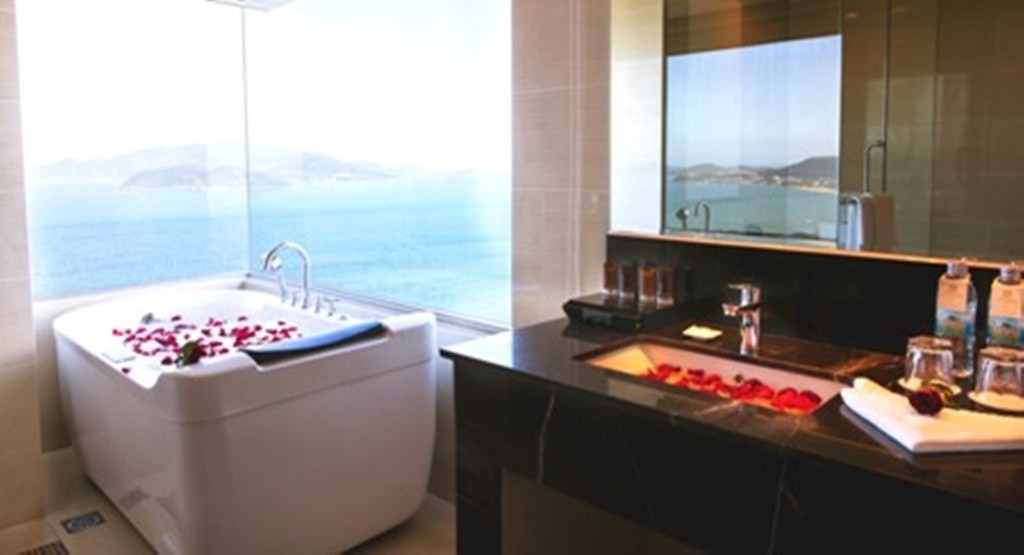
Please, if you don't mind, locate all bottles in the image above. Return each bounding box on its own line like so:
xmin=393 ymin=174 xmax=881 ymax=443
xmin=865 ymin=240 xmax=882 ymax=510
xmin=934 ymin=261 xmax=977 ymax=378
xmin=987 ymin=263 xmax=1024 ymax=348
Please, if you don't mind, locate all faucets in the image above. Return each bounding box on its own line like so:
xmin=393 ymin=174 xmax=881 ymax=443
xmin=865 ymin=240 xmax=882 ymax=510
xmin=721 ymin=282 xmax=763 ymax=356
xmin=694 ymin=201 xmax=711 ymax=229
xmin=260 ymin=239 xmax=314 ymax=309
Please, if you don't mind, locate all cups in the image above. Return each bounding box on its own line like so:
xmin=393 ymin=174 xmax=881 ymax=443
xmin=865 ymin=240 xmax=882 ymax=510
xmin=639 ymin=266 xmax=656 ymax=302
xmin=620 ymin=264 xmax=635 ymax=298
xmin=975 ymin=347 xmax=1024 ymax=405
xmin=603 ymin=262 xmax=619 ymax=294
xmin=656 ymin=268 xmax=673 ymax=306
xmin=903 ymin=335 xmax=953 ymax=387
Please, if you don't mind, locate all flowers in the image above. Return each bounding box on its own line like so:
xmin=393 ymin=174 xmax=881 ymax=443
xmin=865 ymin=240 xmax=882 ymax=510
xmin=907 ymin=387 xmax=945 ymax=418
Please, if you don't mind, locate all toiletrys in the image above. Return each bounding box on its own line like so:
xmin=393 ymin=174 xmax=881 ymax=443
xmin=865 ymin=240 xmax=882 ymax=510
xmin=602 ymin=261 xmax=674 ymax=307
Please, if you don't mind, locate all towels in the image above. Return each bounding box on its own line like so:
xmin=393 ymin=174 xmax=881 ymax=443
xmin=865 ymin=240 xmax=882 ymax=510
xmin=839 ymin=376 xmax=1024 ymax=455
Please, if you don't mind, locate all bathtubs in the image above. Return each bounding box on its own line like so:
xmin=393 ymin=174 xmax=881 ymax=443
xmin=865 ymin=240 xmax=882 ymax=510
xmin=51 ymin=290 xmax=440 ymax=555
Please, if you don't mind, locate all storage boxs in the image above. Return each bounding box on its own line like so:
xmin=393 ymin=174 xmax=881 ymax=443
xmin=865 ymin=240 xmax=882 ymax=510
xmin=562 ymin=290 xmax=685 ymax=335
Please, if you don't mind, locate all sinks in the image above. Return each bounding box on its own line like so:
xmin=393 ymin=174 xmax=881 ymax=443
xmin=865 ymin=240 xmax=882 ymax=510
xmin=570 ymin=333 xmax=852 ymax=415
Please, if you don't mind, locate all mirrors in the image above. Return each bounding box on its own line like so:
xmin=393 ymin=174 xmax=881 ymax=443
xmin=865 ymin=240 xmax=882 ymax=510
xmin=661 ymin=0 xmax=1024 ymax=269
